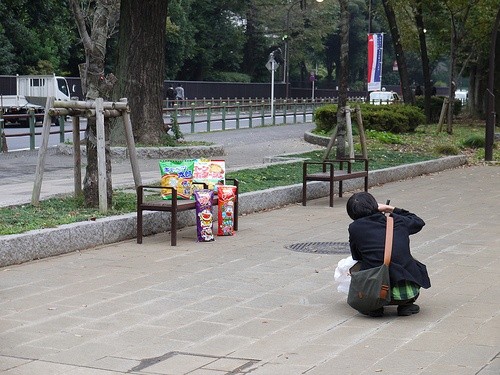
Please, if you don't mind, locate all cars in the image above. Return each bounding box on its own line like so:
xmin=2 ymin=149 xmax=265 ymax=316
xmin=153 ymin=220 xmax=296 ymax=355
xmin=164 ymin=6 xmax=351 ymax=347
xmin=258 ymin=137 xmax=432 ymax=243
xmin=364 ymin=91 xmax=401 ymax=106
xmin=454 ymin=88 xmax=469 ymax=106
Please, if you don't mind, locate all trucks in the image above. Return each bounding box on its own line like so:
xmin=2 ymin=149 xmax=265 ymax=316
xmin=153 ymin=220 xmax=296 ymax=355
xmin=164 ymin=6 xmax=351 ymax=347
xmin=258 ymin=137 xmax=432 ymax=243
xmin=0 ymin=72 xmax=77 ymax=129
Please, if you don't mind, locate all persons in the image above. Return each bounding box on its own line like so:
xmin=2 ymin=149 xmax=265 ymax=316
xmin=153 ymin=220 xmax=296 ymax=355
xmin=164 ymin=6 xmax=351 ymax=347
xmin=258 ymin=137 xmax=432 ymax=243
xmin=348 ymin=191 xmax=432 ymax=315
xmin=166 ymin=84 xmax=176 ymax=109
xmin=176 ymin=84 xmax=185 ymax=114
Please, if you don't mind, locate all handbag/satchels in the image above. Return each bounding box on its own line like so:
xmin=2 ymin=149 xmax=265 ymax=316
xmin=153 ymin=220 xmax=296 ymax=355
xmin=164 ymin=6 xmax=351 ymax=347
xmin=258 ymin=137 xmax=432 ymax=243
xmin=345 ymin=261 xmax=392 ymax=314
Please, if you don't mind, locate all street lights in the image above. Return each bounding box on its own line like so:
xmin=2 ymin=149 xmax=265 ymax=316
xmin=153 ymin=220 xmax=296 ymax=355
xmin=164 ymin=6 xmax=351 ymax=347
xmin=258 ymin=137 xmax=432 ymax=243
xmin=286 ymin=0 xmax=324 ymax=111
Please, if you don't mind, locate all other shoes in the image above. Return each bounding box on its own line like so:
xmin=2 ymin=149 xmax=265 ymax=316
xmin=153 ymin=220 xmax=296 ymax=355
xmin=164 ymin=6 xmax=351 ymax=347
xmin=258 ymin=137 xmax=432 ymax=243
xmin=396 ymin=302 xmax=421 ymax=318
xmin=369 ymin=307 xmax=385 ymax=318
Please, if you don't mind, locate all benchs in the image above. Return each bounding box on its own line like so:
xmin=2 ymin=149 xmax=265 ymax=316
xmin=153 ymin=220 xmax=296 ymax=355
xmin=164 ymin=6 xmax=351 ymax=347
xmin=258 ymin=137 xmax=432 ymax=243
xmin=297 ymin=155 xmax=370 ymax=206
xmin=136 ymin=177 xmax=240 ymax=246
